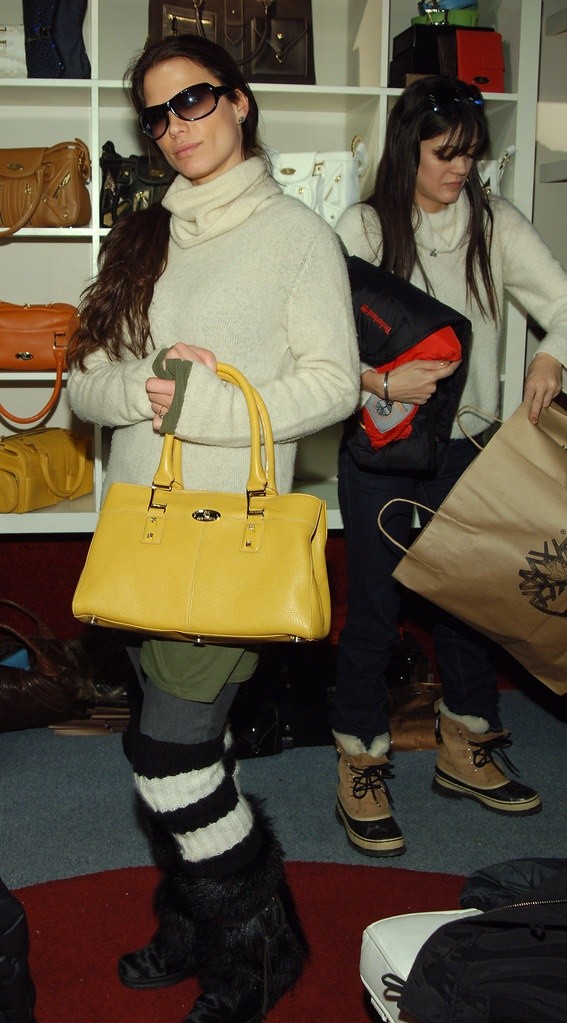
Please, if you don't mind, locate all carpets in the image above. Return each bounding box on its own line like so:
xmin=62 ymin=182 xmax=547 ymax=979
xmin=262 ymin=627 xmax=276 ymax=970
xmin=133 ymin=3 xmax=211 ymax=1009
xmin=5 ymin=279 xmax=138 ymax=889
xmin=7 ymin=859 xmax=469 ymax=1023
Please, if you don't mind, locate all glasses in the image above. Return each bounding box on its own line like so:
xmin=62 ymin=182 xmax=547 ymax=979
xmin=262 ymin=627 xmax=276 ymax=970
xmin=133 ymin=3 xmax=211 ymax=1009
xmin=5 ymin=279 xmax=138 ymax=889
xmin=138 ymin=82 xmax=235 ymax=141
xmin=408 ymin=84 xmax=486 ymax=119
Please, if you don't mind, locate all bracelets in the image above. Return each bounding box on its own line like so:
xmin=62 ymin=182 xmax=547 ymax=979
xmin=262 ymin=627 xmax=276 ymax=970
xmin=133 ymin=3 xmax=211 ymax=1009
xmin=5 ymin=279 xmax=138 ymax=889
xmin=384 ymin=372 xmax=393 ymax=406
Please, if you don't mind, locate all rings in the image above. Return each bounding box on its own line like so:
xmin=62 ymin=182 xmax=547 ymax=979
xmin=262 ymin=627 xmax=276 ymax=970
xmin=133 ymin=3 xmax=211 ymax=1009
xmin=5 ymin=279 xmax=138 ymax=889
xmin=159 ymin=406 xmax=165 ymax=419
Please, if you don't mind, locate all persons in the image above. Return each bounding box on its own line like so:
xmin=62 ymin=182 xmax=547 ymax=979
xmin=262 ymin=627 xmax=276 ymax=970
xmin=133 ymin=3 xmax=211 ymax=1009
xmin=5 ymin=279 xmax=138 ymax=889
xmin=63 ymin=33 xmax=360 ymax=1023
xmin=332 ymin=77 xmax=567 ymax=856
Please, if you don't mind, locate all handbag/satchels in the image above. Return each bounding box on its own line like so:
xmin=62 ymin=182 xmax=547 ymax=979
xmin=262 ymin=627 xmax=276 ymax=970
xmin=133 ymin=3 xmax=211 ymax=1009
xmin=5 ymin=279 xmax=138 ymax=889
xmin=263 ymin=135 xmax=371 ymax=230
xmin=0 ymin=599 xmax=444 ymax=753
xmin=0 ymin=137 xmax=92 ymax=238
xmin=72 ymin=364 xmax=331 ymax=644
xmin=373 ymin=398 xmax=567 ymax=701
xmin=437 ymin=26 xmax=507 ymax=92
xmin=0 ymin=300 xmax=80 ymax=424
xmin=477 ymin=144 xmax=516 ymax=196
xmin=21 ymin=0 xmax=91 ymax=78
xmin=148 ymin=0 xmax=312 ymax=83
xmin=1 ymin=425 xmax=95 ymax=514
xmin=99 ymin=140 xmax=179 ymax=228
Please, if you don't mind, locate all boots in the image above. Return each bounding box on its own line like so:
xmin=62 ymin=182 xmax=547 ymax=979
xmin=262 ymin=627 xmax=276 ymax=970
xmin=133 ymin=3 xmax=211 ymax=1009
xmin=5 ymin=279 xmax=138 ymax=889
xmin=331 ymin=729 xmax=407 ymax=858
xmin=432 ymin=698 xmax=542 ymax=817
xmin=174 ymin=799 xmax=311 ymax=1023
xmin=115 ymin=797 xmax=208 ymax=987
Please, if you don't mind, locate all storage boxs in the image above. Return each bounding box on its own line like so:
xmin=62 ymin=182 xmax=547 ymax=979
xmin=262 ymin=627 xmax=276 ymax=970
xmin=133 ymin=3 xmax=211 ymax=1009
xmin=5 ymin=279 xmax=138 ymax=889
xmin=390 ymin=24 xmax=495 ymax=88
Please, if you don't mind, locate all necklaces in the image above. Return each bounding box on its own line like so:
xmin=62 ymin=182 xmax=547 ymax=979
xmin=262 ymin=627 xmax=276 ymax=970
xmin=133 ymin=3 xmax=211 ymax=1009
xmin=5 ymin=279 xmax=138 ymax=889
xmin=414 ymin=204 xmax=447 ymax=257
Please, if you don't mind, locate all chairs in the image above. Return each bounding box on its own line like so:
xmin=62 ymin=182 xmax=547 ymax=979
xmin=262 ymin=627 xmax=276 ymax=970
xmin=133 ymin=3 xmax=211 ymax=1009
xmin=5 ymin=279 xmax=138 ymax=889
xmin=360 ymin=858 xmax=567 ymax=1023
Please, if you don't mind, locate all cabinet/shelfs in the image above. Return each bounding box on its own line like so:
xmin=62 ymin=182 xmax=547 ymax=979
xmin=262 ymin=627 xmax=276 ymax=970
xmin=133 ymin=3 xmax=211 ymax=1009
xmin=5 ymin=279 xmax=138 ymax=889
xmin=0 ymin=0 xmax=543 ymax=533
